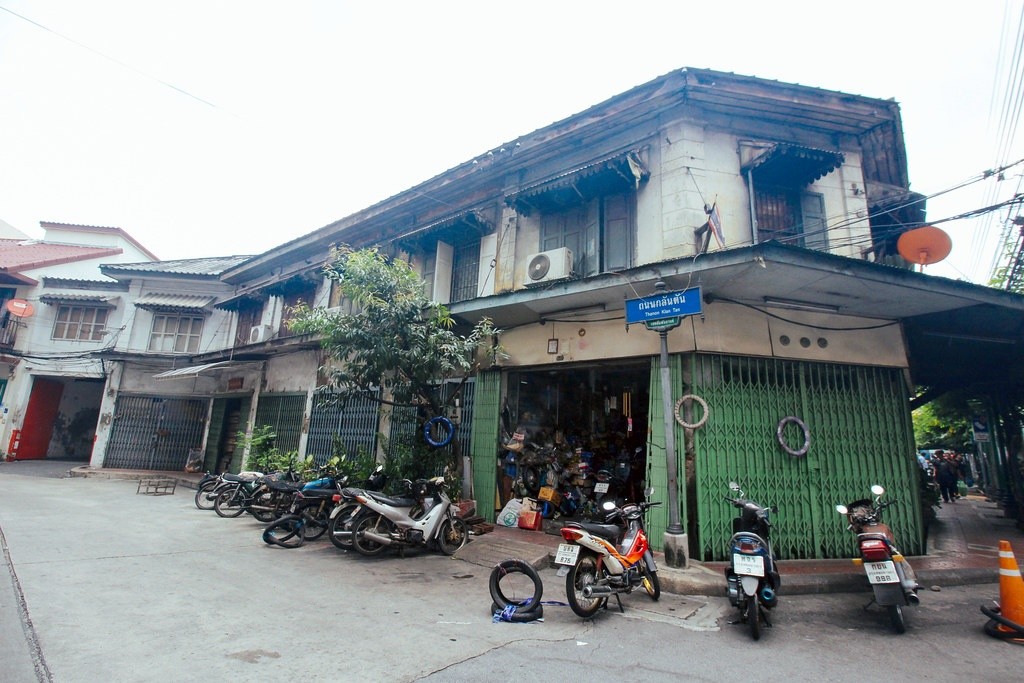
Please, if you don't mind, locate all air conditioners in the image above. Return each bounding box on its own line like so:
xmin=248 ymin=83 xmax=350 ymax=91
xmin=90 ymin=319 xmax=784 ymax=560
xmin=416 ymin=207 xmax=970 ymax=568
xmin=523 ymin=247 xmax=574 ymax=288
xmin=250 ymin=324 xmax=274 ymax=343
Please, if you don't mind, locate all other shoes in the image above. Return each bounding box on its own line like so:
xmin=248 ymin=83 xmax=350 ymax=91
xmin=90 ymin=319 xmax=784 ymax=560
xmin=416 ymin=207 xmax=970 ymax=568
xmin=943 ymin=495 xmax=961 ymax=503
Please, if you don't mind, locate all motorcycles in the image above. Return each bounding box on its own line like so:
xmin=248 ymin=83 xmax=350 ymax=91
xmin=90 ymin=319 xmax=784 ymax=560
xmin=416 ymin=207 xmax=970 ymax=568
xmin=835 ymin=483 xmax=921 ymax=631
xmin=560 ymin=487 xmax=662 ymax=617
xmin=194 ymin=442 xmax=476 ymax=558
xmin=723 ymin=482 xmax=782 ymax=640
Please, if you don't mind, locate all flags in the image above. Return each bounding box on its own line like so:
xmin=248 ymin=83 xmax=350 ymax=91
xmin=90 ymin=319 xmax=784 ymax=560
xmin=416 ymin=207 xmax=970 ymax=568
xmin=708 ymin=203 xmax=726 ymax=250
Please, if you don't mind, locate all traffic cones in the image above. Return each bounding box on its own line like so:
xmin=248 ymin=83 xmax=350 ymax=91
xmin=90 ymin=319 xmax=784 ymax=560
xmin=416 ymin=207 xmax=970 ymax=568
xmin=995 ymin=537 xmax=1024 ymax=642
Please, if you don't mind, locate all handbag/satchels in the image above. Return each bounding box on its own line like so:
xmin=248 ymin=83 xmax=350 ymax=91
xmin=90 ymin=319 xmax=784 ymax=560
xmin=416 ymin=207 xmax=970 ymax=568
xmin=496 ymin=498 xmax=518 ymax=527
xmin=518 ymin=510 xmax=543 ymax=531
